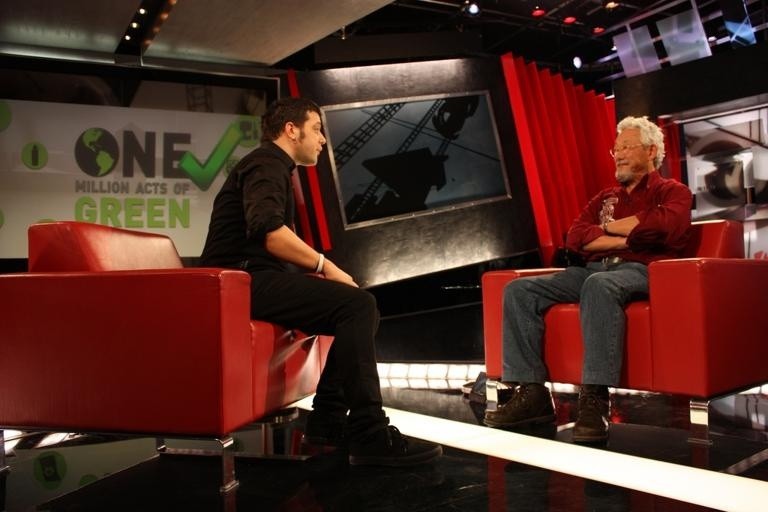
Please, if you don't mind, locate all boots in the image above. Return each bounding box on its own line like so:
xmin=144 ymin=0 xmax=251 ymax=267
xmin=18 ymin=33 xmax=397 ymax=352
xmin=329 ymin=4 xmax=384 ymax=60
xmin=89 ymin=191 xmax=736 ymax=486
xmin=305 ymin=411 xmax=358 ymax=447
xmin=349 ymin=414 xmax=443 ymax=467
xmin=572 ymin=387 xmax=610 ymax=442
xmin=484 ymin=384 xmax=556 ymax=427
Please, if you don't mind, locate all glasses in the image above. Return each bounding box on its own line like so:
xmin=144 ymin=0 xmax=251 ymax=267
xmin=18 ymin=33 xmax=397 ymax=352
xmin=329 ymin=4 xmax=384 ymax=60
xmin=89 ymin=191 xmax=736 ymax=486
xmin=610 ymin=144 xmax=643 ymax=157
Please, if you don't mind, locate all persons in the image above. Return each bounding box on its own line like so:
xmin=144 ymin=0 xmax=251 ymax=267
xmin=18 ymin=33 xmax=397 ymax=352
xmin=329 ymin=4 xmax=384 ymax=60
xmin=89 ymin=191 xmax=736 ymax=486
xmin=198 ymin=95 xmax=444 ymax=467
xmin=482 ymin=116 xmax=694 ymax=446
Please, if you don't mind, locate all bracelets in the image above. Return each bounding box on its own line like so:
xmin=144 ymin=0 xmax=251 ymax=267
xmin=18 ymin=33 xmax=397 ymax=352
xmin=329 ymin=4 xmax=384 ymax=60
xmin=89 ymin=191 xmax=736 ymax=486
xmin=314 ymin=253 xmax=324 ymax=275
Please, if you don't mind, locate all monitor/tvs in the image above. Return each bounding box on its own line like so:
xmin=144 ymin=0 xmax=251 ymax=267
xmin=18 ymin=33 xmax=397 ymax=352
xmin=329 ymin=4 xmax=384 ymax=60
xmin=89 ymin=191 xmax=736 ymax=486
xmin=0 ymin=52 xmax=278 ymax=274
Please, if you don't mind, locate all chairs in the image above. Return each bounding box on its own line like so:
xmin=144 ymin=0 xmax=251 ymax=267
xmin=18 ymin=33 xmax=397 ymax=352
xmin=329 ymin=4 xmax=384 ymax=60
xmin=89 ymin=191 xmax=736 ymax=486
xmin=482 ymin=218 xmax=768 ymax=447
xmin=1 ymin=220 xmax=337 ymax=496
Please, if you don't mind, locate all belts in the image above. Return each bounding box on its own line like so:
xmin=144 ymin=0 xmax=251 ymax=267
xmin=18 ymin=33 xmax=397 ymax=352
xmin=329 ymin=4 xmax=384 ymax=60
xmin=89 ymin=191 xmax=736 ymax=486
xmin=595 ymin=256 xmax=630 ymax=265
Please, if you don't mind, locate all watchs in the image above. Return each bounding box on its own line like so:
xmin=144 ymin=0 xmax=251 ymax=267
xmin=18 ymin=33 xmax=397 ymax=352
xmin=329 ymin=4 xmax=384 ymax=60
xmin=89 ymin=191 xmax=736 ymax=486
xmin=601 ymin=217 xmax=615 ymax=234
